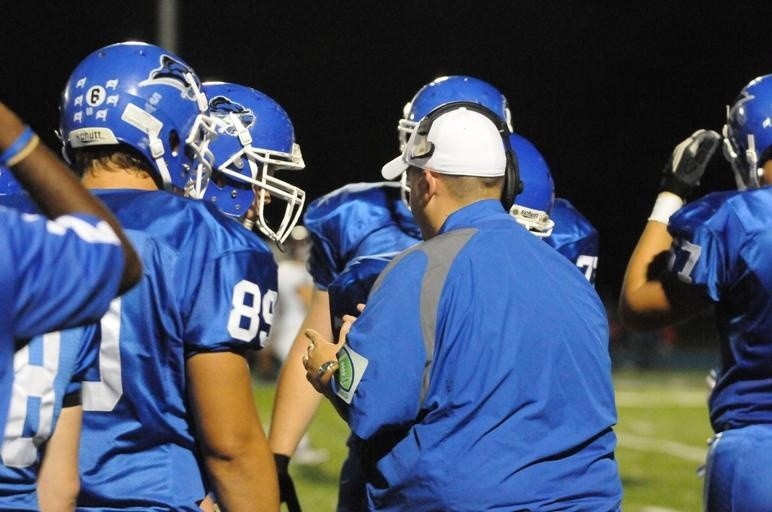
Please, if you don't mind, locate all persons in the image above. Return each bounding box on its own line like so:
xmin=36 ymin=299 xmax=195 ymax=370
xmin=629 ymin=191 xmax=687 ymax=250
xmin=0 ymin=101 xmax=145 ymax=510
xmin=620 ymin=73 xmax=772 ymax=512
xmin=266 ymin=74 xmax=622 ymax=511
xmin=59 ymin=40 xmax=288 ymax=511
xmin=301 ymin=102 xmax=625 ymax=511
xmin=0 ymin=160 xmax=100 ymax=510
xmin=197 ymin=80 xmax=306 ymax=512
xmin=327 ymin=135 xmax=601 ymax=510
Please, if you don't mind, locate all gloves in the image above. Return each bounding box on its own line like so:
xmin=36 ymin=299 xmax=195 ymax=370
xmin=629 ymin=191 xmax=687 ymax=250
xmin=656 ymin=129 xmax=724 ymax=202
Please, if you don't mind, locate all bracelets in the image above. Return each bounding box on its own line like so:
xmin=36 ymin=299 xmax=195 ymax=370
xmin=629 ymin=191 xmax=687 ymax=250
xmin=0 ymin=127 xmax=41 ymax=168
xmin=649 ymin=193 xmax=682 ymax=226
xmin=315 ymin=360 xmax=339 ymax=392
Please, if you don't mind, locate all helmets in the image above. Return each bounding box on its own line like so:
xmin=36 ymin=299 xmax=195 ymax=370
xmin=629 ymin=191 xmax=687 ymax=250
xmin=382 ymin=75 xmax=555 ymax=240
xmin=722 ymin=74 xmax=772 ymax=192
xmin=54 ymin=41 xmax=228 ymax=201
xmin=201 ymin=81 xmax=306 ymax=246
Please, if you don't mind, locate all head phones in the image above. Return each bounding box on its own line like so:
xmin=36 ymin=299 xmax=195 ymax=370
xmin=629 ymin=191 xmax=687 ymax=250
xmin=411 ymin=99 xmax=524 ymax=214
